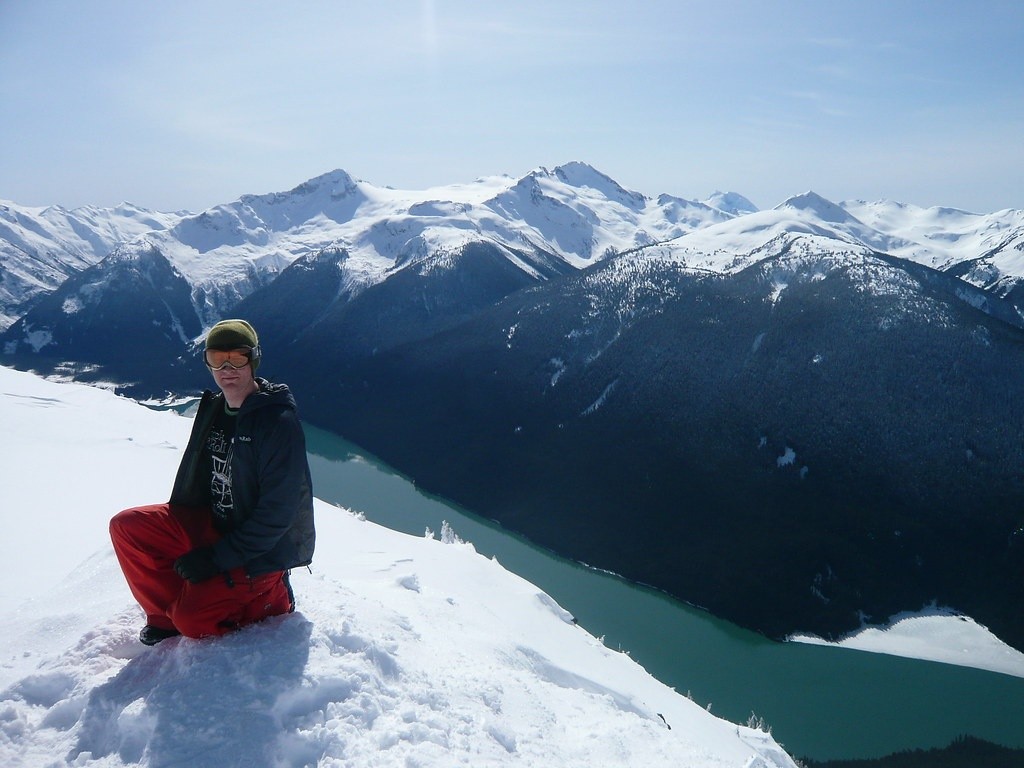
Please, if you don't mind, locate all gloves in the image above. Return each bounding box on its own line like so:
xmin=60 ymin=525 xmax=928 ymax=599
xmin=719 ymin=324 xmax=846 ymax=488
xmin=174 ymin=545 xmax=221 ymax=583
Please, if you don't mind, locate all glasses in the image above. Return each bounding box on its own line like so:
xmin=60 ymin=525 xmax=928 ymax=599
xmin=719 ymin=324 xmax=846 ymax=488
xmin=203 ymin=347 xmax=253 ymax=371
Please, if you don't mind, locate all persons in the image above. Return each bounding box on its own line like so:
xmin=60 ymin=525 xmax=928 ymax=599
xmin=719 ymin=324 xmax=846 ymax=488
xmin=109 ymin=318 xmax=316 ymax=646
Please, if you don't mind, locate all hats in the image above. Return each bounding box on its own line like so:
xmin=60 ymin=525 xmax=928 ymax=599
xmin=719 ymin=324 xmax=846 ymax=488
xmin=204 ymin=318 xmax=260 ymax=371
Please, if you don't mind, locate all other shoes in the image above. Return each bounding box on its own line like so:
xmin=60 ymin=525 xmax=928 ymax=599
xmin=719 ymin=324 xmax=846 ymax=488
xmin=282 ymin=570 xmax=295 ymax=613
xmin=139 ymin=626 xmax=180 ymax=646
